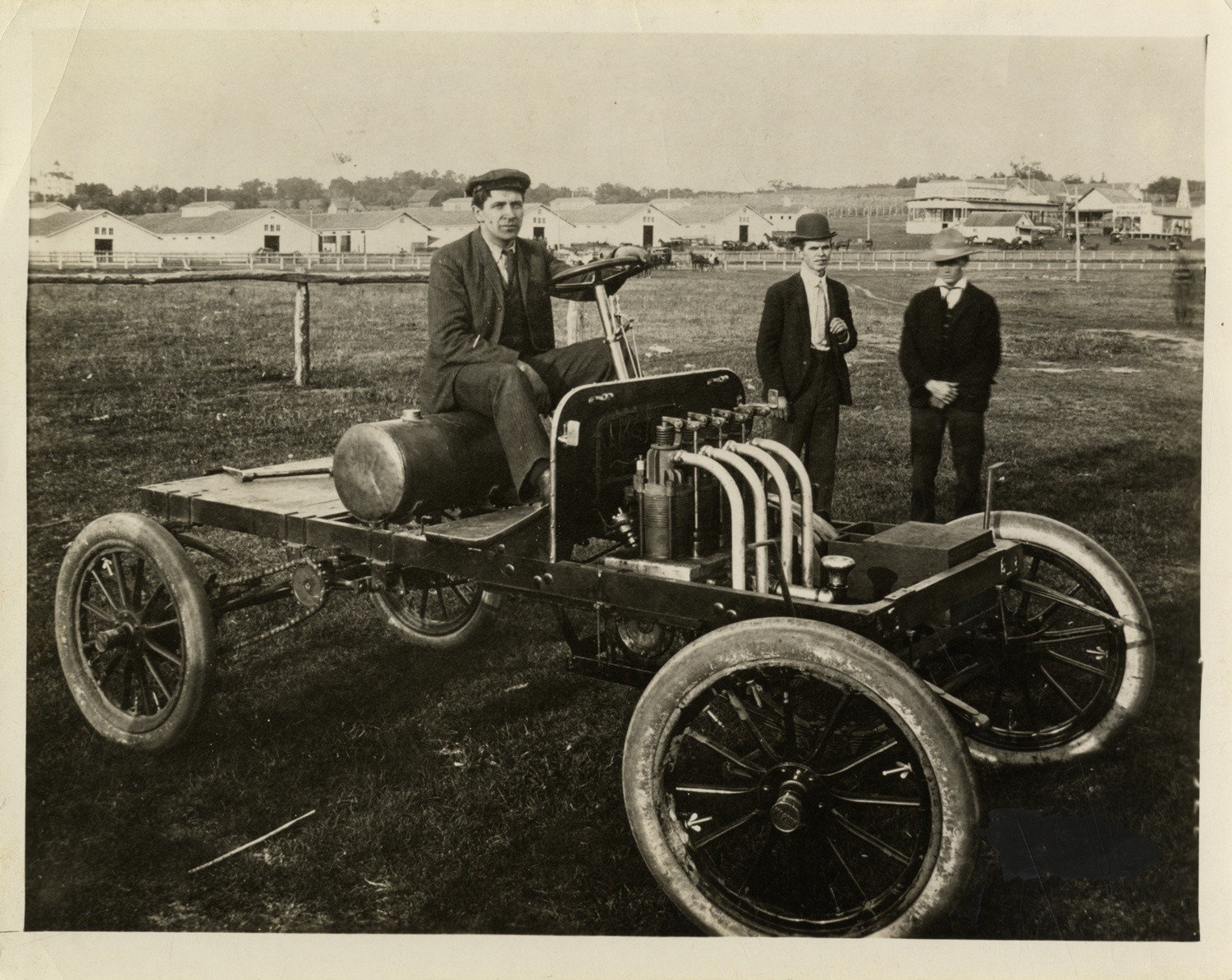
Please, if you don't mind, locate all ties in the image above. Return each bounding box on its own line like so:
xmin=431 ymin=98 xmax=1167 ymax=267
xmin=815 ymin=282 xmax=827 ymax=345
xmin=501 ymin=248 xmax=515 ymax=286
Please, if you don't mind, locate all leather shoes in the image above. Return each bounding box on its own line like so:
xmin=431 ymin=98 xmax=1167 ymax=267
xmin=537 ymin=468 xmax=552 ymax=504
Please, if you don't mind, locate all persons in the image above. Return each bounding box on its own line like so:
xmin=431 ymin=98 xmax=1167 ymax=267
xmin=398 ymin=248 xmax=408 ymax=263
xmin=1169 ymin=255 xmax=1197 ymax=334
xmin=896 ymin=227 xmax=1004 ymax=526
xmin=756 ymin=212 xmax=859 ymax=526
xmin=564 ymin=246 xmax=720 ymax=271
xmin=414 ymin=168 xmax=665 ymax=509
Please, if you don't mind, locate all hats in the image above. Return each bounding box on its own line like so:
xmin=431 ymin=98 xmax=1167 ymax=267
xmin=465 ymin=168 xmax=531 ymax=197
xmin=788 ymin=213 xmax=839 ymax=244
xmin=920 ymin=228 xmax=984 ymax=261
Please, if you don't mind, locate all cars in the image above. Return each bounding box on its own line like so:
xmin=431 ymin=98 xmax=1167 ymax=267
xmin=48 ymin=250 xmax=1157 ymax=938
